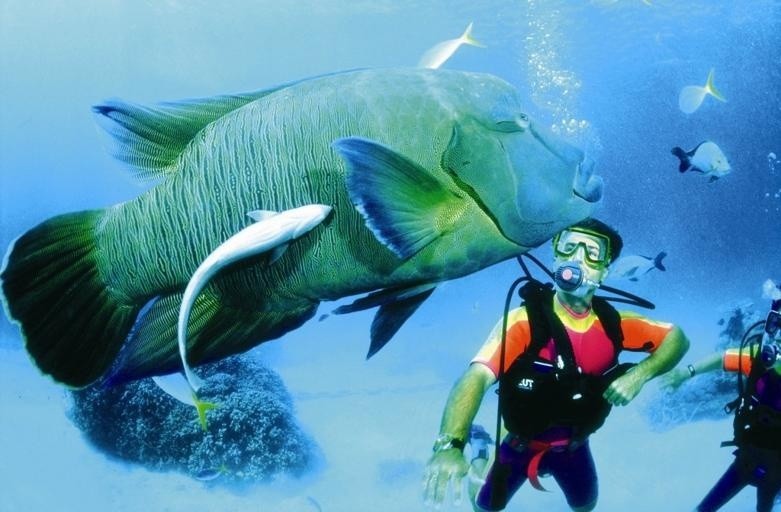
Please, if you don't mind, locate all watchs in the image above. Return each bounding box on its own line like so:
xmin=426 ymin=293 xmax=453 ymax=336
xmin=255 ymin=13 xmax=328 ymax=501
xmin=433 ymin=435 xmax=464 ymax=453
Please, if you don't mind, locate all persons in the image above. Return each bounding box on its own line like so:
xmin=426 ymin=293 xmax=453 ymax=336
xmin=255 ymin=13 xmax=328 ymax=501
xmin=421 ymin=218 xmax=690 ymax=512
xmin=659 ymin=299 xmax=781 ymax=512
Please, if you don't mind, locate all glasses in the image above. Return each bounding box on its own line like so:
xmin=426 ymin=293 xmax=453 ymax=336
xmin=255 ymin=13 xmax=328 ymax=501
xmin=554 ymin=227 xmax=610 ymax=269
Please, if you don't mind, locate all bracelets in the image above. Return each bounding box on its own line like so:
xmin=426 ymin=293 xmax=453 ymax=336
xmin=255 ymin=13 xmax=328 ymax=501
xmin=688 ymin=365 xmax=695 ymax=377
